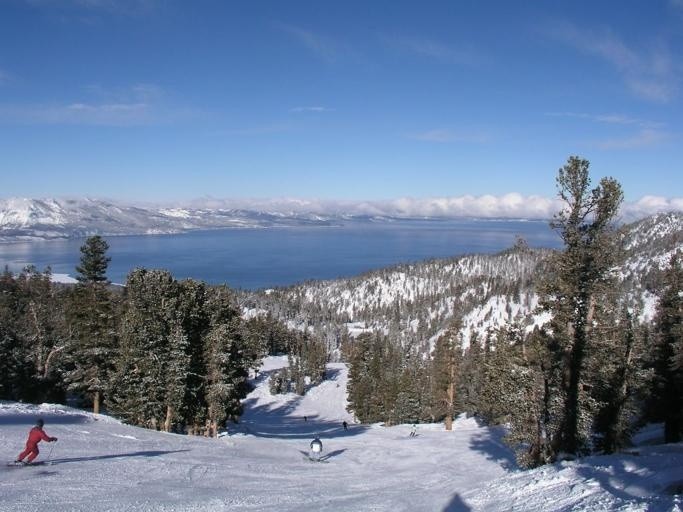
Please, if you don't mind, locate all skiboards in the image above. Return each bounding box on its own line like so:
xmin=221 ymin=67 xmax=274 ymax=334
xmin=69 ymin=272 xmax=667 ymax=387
xmin=7 ymin=461 xmax=58 ymax=466
xmin=305 ymin=456 xmax=328 ymax=464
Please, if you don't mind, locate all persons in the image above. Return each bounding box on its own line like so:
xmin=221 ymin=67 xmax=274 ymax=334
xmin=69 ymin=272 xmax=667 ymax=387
xmin=343 ymin=421 xmax=348 ymax=431
xmin=309 ymin=436 xmax=323 ymax=462
xmin=15 ymin=419 xmax=57 ymax=463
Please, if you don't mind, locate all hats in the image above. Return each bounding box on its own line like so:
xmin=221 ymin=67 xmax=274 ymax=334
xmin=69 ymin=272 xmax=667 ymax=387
xmin=37 ymin=420 xmax=43 ymax=426
